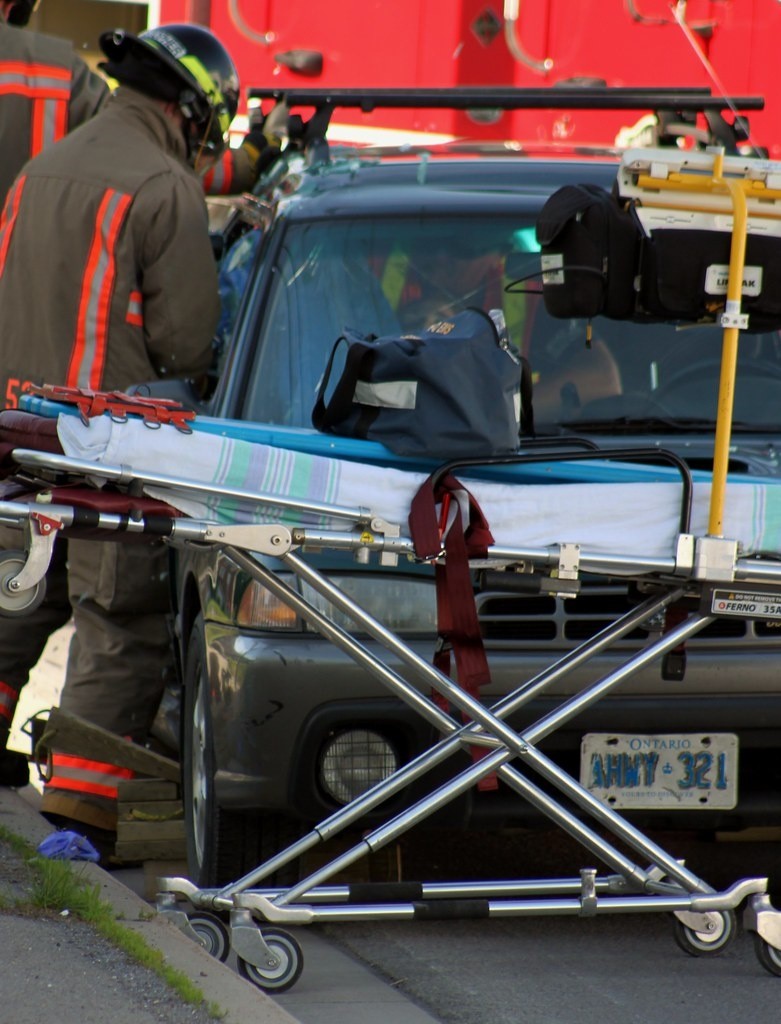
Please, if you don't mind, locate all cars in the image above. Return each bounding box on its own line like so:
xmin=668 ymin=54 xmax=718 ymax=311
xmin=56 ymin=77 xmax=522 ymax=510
xmin=169 ymin=160 xmax=781 ymax=890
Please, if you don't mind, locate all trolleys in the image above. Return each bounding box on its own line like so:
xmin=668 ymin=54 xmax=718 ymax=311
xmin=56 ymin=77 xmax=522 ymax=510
xmin=0 ymin=380 xmax=781 ymax=991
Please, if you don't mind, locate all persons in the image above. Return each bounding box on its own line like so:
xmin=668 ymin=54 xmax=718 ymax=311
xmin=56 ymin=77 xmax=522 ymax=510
xmin=396 ymin=249 xmax=623 ymax=423
xmin=0 ymin=0 xmax=282 ymax=870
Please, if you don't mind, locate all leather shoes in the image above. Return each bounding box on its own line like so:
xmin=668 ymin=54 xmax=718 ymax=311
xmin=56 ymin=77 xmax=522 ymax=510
xmin=1 ymin=749 xmax=29 ymax=789
xmin=53 ymin=813 xmax=144 ymax=869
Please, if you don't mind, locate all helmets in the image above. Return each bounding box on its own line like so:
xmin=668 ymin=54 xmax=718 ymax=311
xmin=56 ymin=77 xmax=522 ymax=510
xmin=98 ymin=23 xmax=240 ymax=162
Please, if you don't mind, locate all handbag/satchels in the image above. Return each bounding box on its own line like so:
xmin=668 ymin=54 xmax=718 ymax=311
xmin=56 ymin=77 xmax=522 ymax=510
xmin=311 ymin=306 xmax=533 ymax=461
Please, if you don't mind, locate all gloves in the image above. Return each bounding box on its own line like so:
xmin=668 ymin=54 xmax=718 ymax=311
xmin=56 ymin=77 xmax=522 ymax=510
xmin=243 ymin=130 xmax=281 ymax=179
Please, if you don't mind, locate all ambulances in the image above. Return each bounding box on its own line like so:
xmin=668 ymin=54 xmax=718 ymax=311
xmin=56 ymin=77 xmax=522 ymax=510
xmin=2 ymin=0 xmax=781 ymax=251
